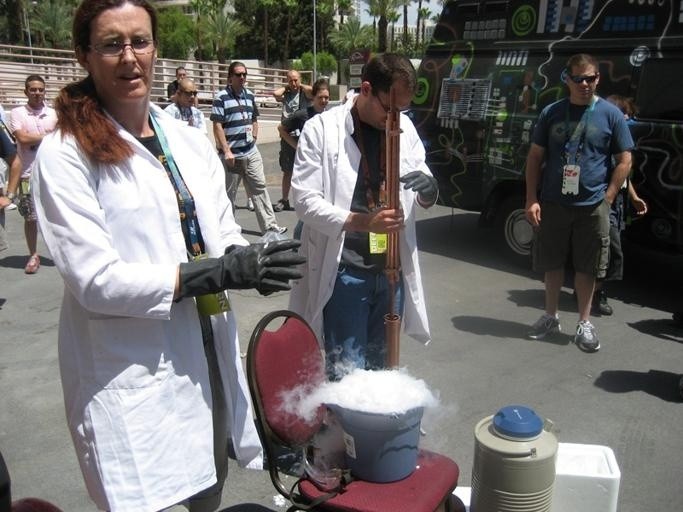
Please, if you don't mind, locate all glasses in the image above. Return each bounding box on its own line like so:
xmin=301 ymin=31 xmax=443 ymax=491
xmin=374 ymin=93 xmax=410 ymax=115
xmin=233 ymin=72 xmax=247 ymax=77
xmin=568 ymin=73 xmax=600 ymax=85
xmin=183 ymin=91 xmax=198 ymax=96
xmin=86 ymin=37 xmax=158 ymax=57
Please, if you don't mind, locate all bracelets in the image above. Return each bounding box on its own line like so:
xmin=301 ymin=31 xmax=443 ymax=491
xmin=5 ymin=191 xmax=17 ymax=203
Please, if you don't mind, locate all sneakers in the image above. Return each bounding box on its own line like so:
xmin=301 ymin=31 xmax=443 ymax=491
xmin=575 ymin=318 xmax=602 ymax=354
xmin=247 ymin=201 xmax=255 ymax=212
xmin=596 ymin=288 xmax=613 ymax=317
xmin=271 ymin=224 xmax=287 ymax=235
xmin=275 ymin=199 xmax=290 ymax=212
xmin=526 ymin=311 xmax=562 ymax=340
xmin=24 ymin=254 xmax=42 ymax=275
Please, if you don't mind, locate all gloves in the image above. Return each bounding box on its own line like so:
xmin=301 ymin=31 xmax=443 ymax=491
xmin=177 ymin=238 xmax=308 ymax=296
xmin=398 ymin=170 xmax=440 ymax=202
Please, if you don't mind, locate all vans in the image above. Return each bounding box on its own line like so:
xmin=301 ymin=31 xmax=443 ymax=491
xmin=405 ymin=0 xmax=682 ymax=265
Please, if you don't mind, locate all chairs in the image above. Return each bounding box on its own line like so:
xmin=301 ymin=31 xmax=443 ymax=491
xmin=247 ymin=310 xmax=459 ymax=512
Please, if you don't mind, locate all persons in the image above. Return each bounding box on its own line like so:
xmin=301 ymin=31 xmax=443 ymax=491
xmin=287 ymin=53 xmax=439 ymax=380
xmin=590 ymin=94 xmax=649 ymax=316
xmin=522 ymin=53 xmax=635 ymax=352
xmin=0 ymin=105 xmax=13 ymax=251
xmin=29 ymin=0 xmax=306 ymax=512
xmin=9 ymin=74 xmax=60 ymax=274
xmin=0 ymin=118 xmax=22 ymax=212
xmin=165 ymin=62 xmax=332 ymax=251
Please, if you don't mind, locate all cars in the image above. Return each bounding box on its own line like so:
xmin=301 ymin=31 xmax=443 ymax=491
xmin=253 ymin=95 xmax=285 ymax=108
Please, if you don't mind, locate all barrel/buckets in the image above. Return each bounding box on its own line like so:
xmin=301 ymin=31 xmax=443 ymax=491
xmin=324 ymin=402 xmax=425 ymax=483
xmin=468 ymin=405 xmax=560 ymax=512
